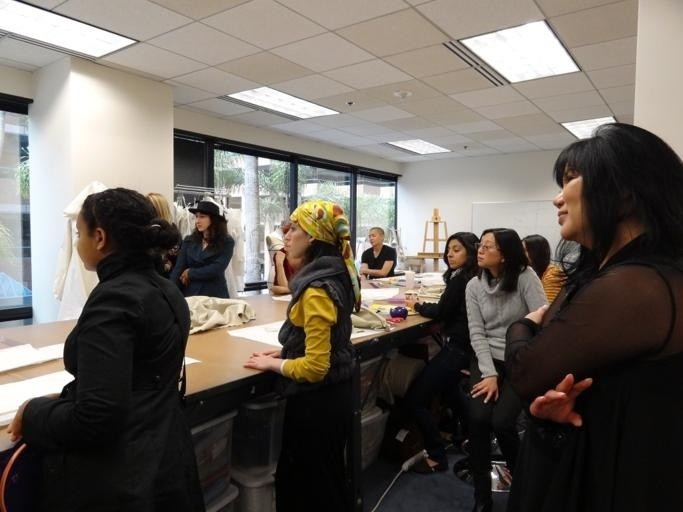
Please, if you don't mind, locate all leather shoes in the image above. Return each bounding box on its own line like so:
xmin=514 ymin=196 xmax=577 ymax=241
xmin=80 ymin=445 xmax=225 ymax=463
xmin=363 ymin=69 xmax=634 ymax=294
xmin=472 ymin=496 xmax=493 ymax=512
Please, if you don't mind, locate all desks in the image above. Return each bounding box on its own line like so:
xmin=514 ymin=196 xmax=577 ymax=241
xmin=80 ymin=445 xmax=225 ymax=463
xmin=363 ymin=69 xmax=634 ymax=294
xmin=0 ymin=271 xmax=505 ymax=511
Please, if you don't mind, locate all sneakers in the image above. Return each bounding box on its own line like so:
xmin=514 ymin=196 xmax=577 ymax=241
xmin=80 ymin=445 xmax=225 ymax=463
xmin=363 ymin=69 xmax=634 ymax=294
xmin=413 ymin=455 xmax=449 ymax=475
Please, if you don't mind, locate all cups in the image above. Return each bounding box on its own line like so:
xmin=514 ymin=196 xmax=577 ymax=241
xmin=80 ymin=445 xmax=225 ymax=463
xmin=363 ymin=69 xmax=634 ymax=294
xmin=404 ymin=292 xmax=419 ymax=313
xmin=405 ymin=271 xmax=415 ymax=290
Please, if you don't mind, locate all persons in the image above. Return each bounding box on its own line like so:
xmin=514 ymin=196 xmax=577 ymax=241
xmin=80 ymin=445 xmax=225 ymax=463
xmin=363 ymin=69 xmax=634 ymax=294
xmin=242 ymin=198 xmax=363 ymax=512
xmin=167 ymin=200 xmax=234 ymax=300
xmin=356 ymin=226 xmax=399 ymax=280
xmin=268 ymin=224 xmax=294 ymax=294
xmin=145 ymin=191 xmax=181 ymax=275
xmin=503 ymin=122 xmax=683 ymax=510
xmin=7 ymin=186 xmax=205 ymax=510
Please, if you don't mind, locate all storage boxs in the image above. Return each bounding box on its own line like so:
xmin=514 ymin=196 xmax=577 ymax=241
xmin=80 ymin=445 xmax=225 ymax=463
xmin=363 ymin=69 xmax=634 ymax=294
xmin=353 ymin=346 xmax=394 ymax=471
xmin=187 ymin=394 xmax=280 ymax=512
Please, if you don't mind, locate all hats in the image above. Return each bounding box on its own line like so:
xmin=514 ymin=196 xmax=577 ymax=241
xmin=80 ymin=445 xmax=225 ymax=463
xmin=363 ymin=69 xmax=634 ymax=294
xmin=189 ymin=201 xmax=219 ymax=217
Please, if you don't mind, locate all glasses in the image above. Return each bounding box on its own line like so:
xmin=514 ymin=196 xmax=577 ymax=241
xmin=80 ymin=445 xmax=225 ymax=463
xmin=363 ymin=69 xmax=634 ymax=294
xmin=474 ymin=242 xmax=500 ymax=251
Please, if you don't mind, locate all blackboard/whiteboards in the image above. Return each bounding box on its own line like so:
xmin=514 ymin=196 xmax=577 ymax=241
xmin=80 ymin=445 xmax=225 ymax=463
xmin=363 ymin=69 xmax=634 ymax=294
xmin=472 ymin=201 xmax=561 ymax=262
